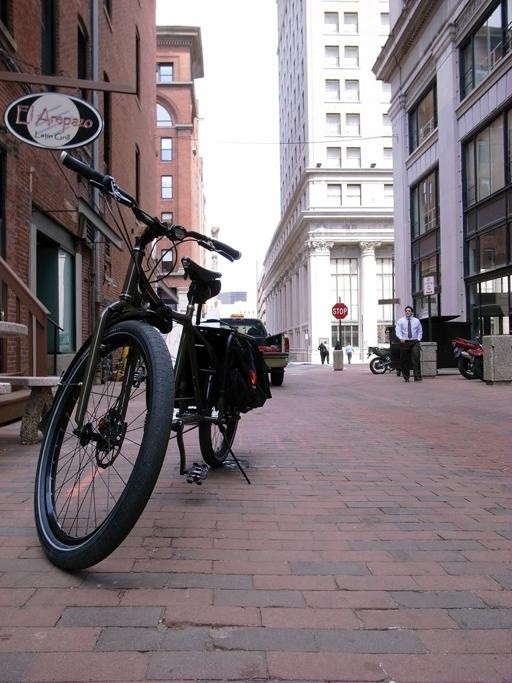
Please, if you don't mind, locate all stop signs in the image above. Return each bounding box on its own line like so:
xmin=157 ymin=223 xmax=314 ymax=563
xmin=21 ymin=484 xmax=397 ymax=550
xmin=332 ymin=303 xmax=348 ymax=319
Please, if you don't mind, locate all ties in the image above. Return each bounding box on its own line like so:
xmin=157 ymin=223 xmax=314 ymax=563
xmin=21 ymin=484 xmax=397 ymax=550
xmin=407 ymin=318 xmax=413 ymax=339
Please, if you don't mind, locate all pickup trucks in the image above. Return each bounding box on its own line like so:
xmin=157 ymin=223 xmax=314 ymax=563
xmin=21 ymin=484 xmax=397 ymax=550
xmin=215 ymin=312 xmax=291 ymax=386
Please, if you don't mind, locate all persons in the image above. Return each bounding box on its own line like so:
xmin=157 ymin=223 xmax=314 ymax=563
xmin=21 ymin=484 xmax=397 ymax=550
xmin=395 ymin=304 xmax=424 ymax=382
xmin=318 ymin=342 xmax=327 ymax=364
xmin=322 ymin=339 xmax=330 ymax=364
xmin=345 ymin=342 xmax=355 ymax=364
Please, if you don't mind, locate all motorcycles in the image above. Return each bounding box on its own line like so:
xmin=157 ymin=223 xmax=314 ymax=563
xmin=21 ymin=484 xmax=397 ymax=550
xmin=366 ymin=347 xmax=395 ymax=375
xmin=451 ymin=336 xmax=484 ymax=382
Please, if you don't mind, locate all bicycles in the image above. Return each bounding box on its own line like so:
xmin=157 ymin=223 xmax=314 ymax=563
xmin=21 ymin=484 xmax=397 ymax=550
xmin=32 ymin=152 xmax=251 ymax=572
